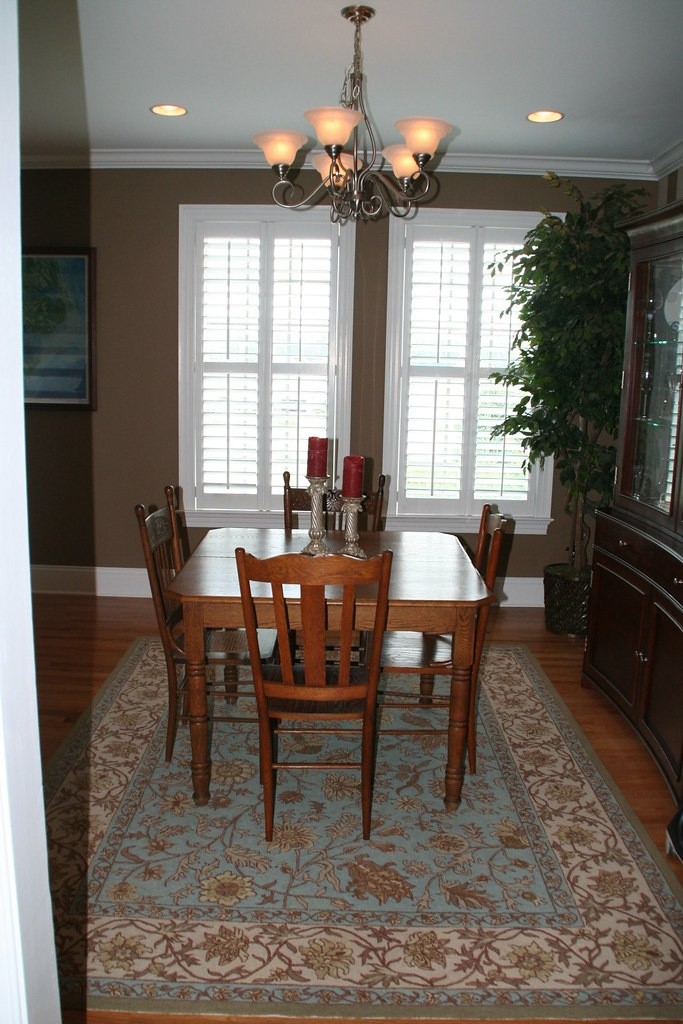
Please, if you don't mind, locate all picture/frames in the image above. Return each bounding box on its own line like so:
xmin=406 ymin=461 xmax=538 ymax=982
xmin=23 ymin=245 xmax=99 ymax=411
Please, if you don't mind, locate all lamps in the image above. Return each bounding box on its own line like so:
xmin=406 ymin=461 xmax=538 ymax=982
xmin=252 ymin=5 xmax=452 ymax=224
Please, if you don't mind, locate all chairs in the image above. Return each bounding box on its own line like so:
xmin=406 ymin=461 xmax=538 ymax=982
xmin=235 ymin=547 xmax=393 ymax=843
xmin=133 ymin=485 xmax=278 ymax=762
xmin=283 ymin=471 xmax=386 ymax=530
xmin=360 ymin=504 xmax=516 ymax=773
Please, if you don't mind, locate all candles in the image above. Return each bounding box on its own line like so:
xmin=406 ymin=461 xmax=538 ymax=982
xmin=342 ymin=455 xmax=364 ymax=498
xmin=307 ymin=436 xmax=329 ymax=476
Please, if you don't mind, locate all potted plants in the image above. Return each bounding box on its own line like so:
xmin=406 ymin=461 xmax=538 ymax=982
xmin=486 ymin=172 xmax=650 ymax=633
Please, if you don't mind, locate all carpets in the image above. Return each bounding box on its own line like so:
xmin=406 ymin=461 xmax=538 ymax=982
xmin=44 ymin=634 xmax=683 ymax=1020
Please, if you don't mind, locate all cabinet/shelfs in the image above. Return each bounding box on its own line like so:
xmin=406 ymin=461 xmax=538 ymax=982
xmin=580 ymin=194 xmax=683 ymax=807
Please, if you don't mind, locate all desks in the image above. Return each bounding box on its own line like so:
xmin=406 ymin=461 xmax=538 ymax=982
xmin=159 ymin=527 xmax=496 ymax=810
